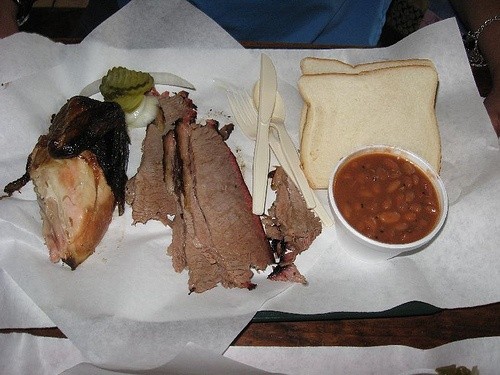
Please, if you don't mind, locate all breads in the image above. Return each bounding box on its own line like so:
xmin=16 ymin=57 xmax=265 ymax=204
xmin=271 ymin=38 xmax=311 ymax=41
xmin=296 ymin=57 xmax=441 ymax=187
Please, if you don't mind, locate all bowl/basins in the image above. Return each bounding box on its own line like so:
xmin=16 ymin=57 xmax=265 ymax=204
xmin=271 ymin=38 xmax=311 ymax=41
xmin=328 ymin=144 xmax=448 ymax=255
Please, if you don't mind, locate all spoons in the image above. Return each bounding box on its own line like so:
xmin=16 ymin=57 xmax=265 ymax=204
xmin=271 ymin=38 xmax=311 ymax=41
xmin=252 ymin=80 xmax=316 ymax=209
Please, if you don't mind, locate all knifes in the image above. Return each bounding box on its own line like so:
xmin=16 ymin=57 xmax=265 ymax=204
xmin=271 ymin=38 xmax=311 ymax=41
xmin=251 ymin=53 xmax=278 ymax=214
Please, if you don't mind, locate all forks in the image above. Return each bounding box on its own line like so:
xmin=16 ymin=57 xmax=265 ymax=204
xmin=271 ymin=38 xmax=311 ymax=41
xmin=226 ymin=87 xmax=334 ymax=228
